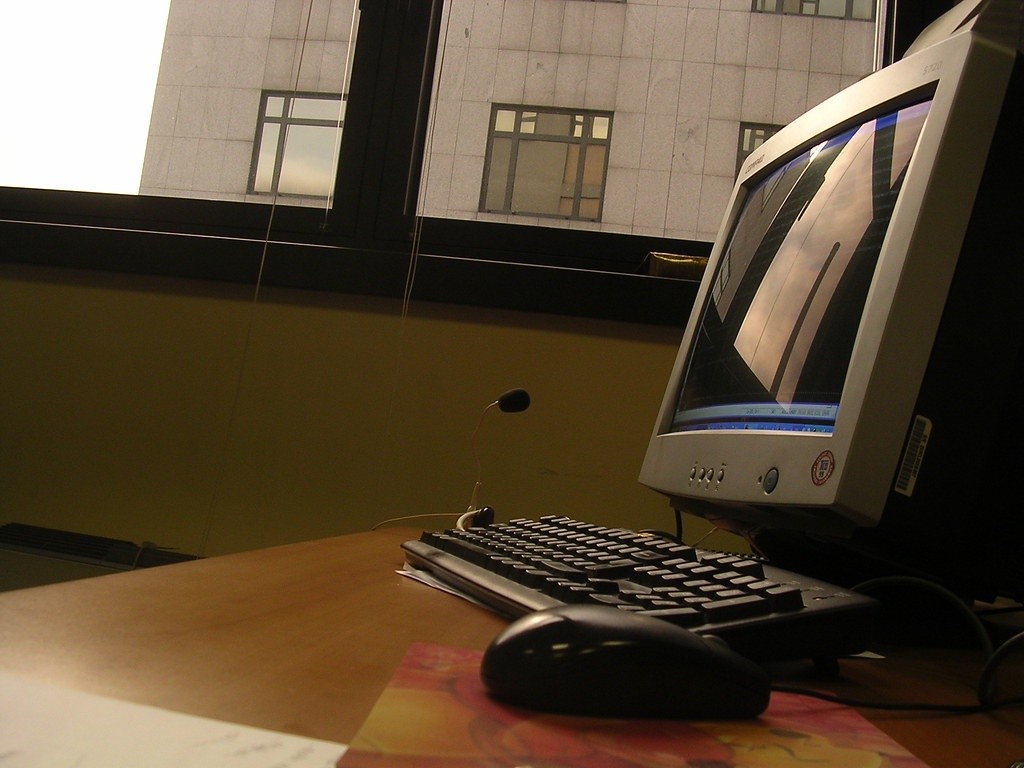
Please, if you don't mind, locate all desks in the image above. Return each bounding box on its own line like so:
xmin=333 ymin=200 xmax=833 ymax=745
xmin=0 ymin=527 xmax=1024 ymax=768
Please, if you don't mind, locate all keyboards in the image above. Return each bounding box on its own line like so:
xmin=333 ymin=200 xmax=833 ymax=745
xmin=402 ymin=513 xmax=881 ymax=659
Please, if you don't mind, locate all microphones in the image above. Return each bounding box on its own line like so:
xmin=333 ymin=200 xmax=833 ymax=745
xmin=468 ymin=390 xmax=532 ymax=513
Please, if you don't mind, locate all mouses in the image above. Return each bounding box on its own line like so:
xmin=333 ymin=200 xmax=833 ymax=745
xmin=480 ymin=603 xmax=770 ymax=723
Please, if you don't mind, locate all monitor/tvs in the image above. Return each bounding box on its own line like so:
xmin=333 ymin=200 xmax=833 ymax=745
xmin=639 ymin=27 xmax=1024 ymax=632
